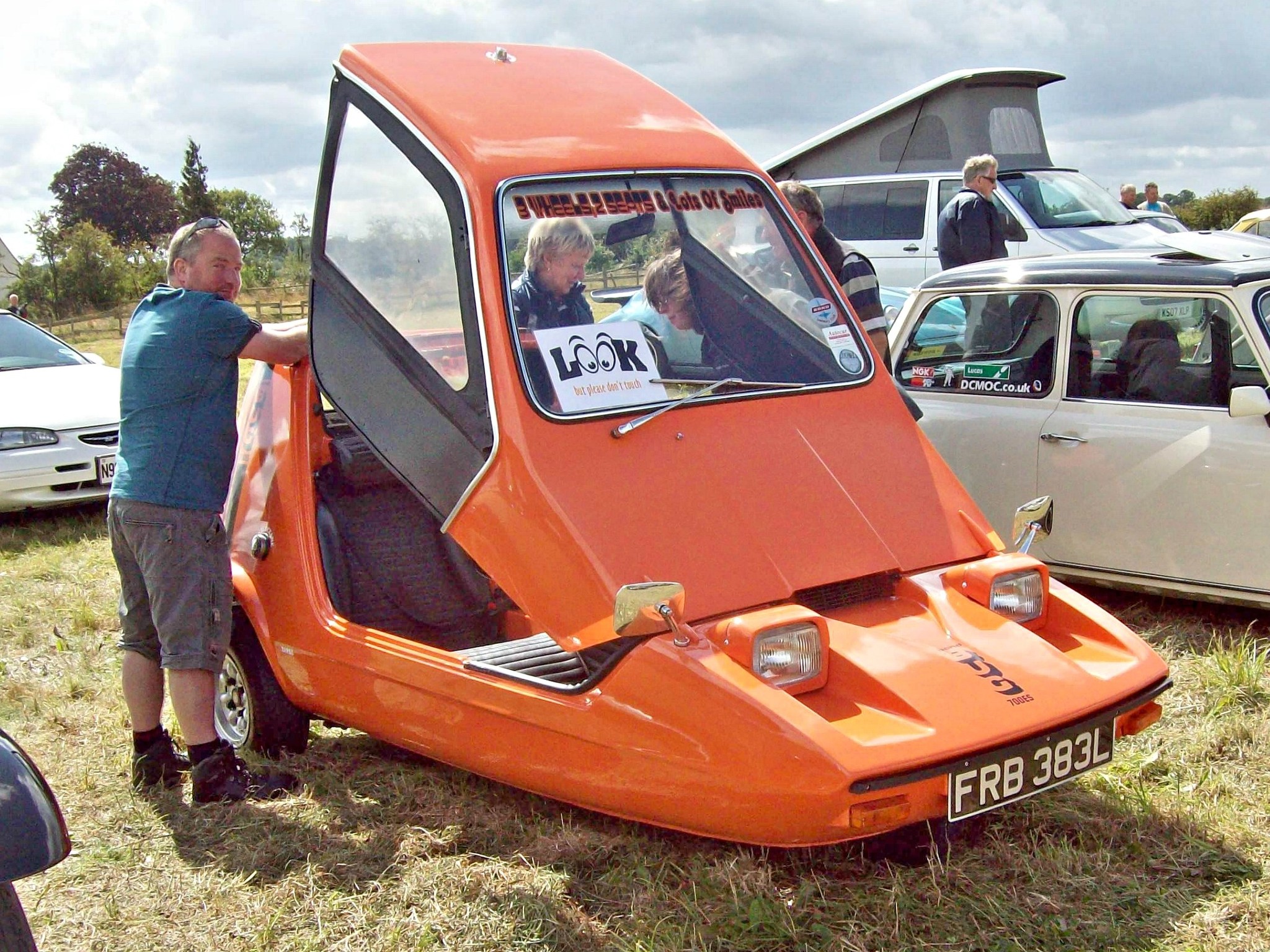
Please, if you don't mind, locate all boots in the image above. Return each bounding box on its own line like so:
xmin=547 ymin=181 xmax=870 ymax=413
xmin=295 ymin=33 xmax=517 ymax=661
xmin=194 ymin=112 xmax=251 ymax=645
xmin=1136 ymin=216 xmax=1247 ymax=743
xmin=190 ymin=740 xmax=299 ymax=803
xmin=132 ymin=729 xmax=191 ymax=788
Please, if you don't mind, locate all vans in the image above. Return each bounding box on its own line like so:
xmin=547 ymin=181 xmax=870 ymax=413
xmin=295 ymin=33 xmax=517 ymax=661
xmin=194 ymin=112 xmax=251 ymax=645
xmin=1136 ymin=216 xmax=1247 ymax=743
xmin=727 ymin=67 xmax=1204 ymax=339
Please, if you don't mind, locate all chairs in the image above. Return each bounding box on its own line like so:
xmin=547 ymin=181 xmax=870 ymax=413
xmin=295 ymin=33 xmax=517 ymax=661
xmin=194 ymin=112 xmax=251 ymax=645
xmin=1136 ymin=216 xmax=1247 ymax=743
xmin=1114 ymin=321 xmax=1180 ymax=403
xmin=1027 ymin=333 xmax=1095 ymax=399
xmin=331 ymin=435 xmax=503 ymax=652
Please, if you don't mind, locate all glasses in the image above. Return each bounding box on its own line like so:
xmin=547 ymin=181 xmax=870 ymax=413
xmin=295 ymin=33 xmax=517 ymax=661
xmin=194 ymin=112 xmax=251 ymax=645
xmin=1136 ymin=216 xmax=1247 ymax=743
xmin=981 ymin=176 xmax=996 ymax=184
xmin=648 ymin=285 xmax=685 ymax=308
xmin=174 ymin=216 xmax=233 ymax=258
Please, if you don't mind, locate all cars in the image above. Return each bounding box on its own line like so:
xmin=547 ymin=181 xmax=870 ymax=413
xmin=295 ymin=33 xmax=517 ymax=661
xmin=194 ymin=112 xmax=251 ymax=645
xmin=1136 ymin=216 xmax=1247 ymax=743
xmin=0 ymin=308 xmax=122 ymax=513
xmin=208 ymin=43 xmax=1174 ymax=868
xmin=876 ymin=285 xmax=968 ymax=344
xmin=886 ymin=228 xmax=1270 ymax=610
xmin=1226 ymin=208 xmax=1270 ymax=239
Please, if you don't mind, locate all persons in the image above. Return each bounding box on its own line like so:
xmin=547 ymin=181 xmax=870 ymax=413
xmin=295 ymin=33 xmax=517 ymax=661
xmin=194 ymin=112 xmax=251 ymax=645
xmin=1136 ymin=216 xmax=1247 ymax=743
xmin=1118 ymin=184 xmax=1138 ymax=209
xmin=106 ymin=217 xmax=307 ymax=804
xmin=937 ymin=153 xmax=1011 ymax=351
xmin=645 ymin=245 xmax=828 ymax=363
xmin=7 ymin=294 xmax=27 ymax=319
xmin=511 ymin=217 xmax=596 ymax=412
xmin=760 ymin=181 xmax=893 ymax=376
xmin=597 ymin=211 xmax=736 ymax=361
xmin=1137 ymin=182 xmax=1174 ymax=217
